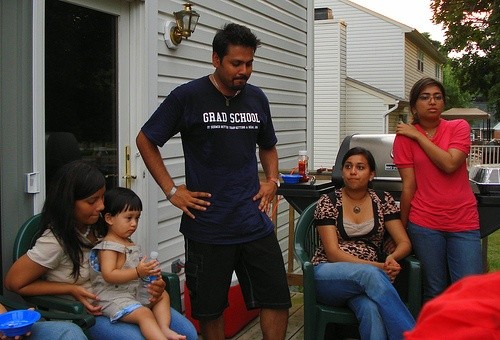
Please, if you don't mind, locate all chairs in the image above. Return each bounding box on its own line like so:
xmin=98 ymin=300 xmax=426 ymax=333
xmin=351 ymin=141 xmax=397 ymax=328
xmin=0 ymin=213 xmax=183 ymax=330
xmin=292 ymin=202 xmax=422 ymax=340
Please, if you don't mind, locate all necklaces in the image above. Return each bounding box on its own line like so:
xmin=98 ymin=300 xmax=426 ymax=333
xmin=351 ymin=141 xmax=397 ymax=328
xmin=424 ymin=127 xmax=433 ymax=138
xmin=211 ymin=73 xmax=241 ymax=106
xmin=345 ymin=186 xmax=367 ymax=213
xmin=73 ymin=224 xmax=91 ymax=238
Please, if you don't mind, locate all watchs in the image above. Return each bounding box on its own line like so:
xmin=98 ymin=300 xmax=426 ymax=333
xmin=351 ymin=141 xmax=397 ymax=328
xmin=266 ymin=176 xmax=281 ymax=191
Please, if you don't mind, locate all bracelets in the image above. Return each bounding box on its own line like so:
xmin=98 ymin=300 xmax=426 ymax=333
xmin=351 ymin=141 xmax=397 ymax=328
xmin=166 ymin=185 xmax=178 ymax=200
xmin=136 ymin=267 xmax=147 ymax=282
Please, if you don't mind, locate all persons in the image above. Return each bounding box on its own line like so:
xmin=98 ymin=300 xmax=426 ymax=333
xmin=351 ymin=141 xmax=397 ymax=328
xmin=5 ymin=161 xmax=199 ymax=340
xmin=311 ymin=148 xmax=417 ymax=340
xmin=136 ymin=22 xmax=292 ymax=340
xmin=88 ymin=187 xmax=187 ymax=340
xmin=393 ymin=77 xmax=483 ymax=308
xmin=0 ymin=303 xmax=88 ymax=340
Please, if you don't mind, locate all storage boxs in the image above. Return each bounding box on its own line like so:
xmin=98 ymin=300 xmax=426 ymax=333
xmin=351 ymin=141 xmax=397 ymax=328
xmin=184 ymin=271 xmax=261 ymax=338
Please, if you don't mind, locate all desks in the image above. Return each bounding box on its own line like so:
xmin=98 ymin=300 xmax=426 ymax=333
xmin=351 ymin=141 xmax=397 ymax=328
xmin=272 ymin=179 xmax=335 ymax=287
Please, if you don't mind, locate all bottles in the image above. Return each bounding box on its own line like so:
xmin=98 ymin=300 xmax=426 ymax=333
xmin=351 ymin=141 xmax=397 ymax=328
xmin=297 ymin=150 xmax=310 ymax=182
xmin=135 ymin=251 xmax=160 ymax=305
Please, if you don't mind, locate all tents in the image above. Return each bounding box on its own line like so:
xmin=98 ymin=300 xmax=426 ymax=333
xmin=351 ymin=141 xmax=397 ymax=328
xmin=440 ymin=107 xmax=492 ymax=144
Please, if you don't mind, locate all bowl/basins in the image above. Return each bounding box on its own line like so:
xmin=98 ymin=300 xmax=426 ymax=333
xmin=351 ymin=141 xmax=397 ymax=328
xmin=1 ymin=310 xmax=42 ymax=338
xmin=281 ymin=174 xmax=303 ymax=185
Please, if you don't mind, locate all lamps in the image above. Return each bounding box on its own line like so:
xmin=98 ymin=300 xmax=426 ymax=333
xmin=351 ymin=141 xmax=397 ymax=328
xmin=164 ymin=0 xmax=200 ymax=50
